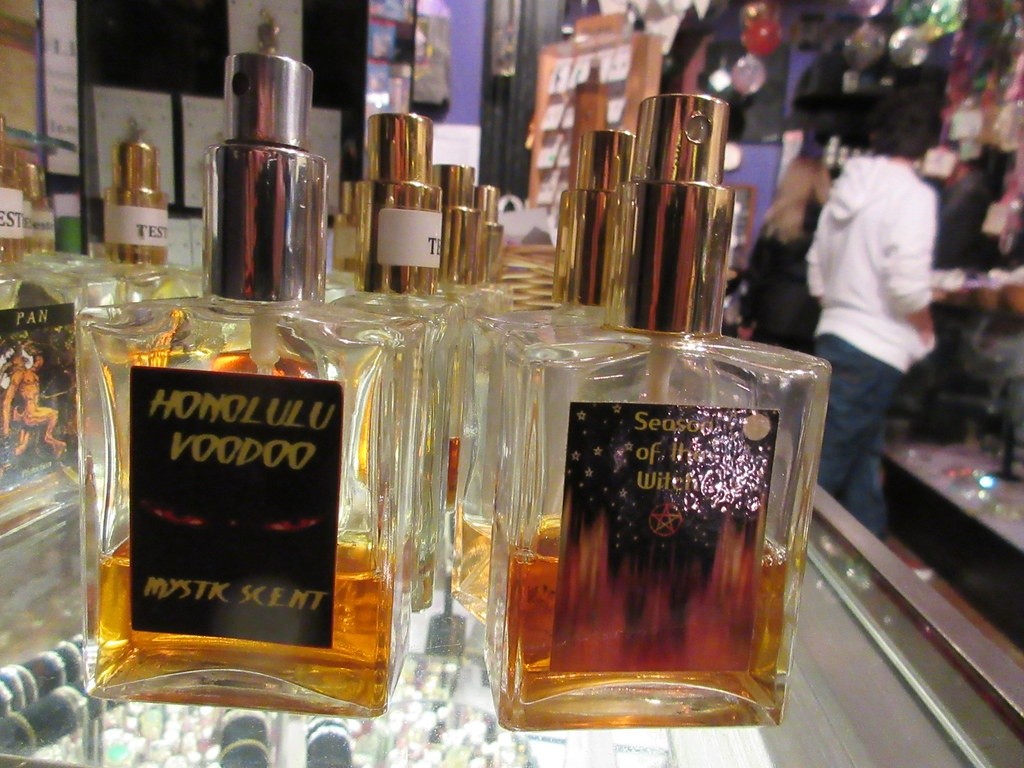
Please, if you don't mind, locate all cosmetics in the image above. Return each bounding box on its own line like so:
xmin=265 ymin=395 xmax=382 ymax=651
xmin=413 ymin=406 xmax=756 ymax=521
xmin=0 ymin=51 xmax=832 ymax=734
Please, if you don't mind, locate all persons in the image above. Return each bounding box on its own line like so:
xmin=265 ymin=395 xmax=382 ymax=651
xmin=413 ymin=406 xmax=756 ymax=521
xmin=741 ymin=93 xmax=1006 ymax=536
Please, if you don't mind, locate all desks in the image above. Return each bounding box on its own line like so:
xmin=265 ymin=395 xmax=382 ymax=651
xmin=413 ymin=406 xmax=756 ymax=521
xmin=875 ymin=436 xmax=1024 ymax=652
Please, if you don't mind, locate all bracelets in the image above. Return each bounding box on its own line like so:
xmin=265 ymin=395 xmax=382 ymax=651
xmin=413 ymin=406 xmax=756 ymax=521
xmin=0 ymin=635 xmax=107 ymax=755
xmin=308 ymin=726 xmax=354 ymax=752
xmin=219 ymin=739 xmax=269 ymax=763
xmin=222 ymin=709 xmax=270 ymax=737
xmin=308 ymin=719 xmax=349 ymax=730
